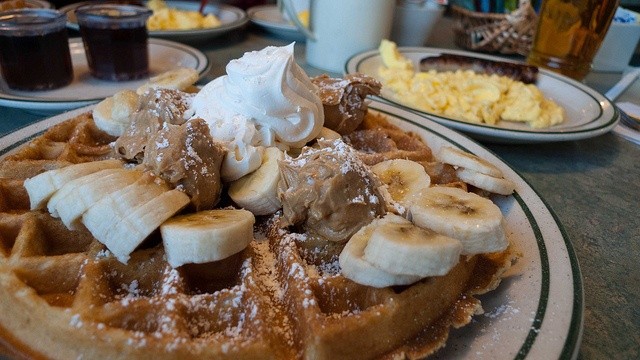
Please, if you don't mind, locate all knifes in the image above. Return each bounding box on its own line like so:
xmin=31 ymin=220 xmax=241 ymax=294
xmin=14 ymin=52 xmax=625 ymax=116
xmin=604 ymin=66 xmax=639 ymax=102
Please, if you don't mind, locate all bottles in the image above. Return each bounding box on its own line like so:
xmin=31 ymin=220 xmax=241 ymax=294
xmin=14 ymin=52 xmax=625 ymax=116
xmin=530 ymin=2 xmax=609 ymax=77
xmin=307 ymin=1 xmax=395 ymax=75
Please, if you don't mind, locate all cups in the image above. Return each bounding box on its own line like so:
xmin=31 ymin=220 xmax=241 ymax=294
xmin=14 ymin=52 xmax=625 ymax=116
xmin=76 ymin=7 xmax=157 ymax=80
xmin=390 ymin=5 xmax=445 ymax=47
xmin=0 ymin=10 xmax=75 ymax=92
xmin=593 ymin=12 xmax=640 ymax=72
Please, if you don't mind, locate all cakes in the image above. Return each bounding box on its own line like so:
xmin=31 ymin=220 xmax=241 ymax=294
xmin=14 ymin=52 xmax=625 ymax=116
xmin=1 ymin=40 xmax=520 ymax=359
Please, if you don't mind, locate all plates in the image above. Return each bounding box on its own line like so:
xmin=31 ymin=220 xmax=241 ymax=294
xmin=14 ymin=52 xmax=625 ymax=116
xmin=140 ymin=4 xmax=248 ymax=42
xmin=1 ymin=38 xmax=213 ymax=110
xmin=0 ymin=95 xmax=583 ymax=360
xmin=242 ymin=6 xmax=301 ymax=39
xmin=342 ymin=46 xmax=622 ymax=142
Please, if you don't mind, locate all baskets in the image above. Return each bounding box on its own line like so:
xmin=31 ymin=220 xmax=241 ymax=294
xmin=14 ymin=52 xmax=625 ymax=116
xmin=450 ymin=1 xmax=538 ymax=56
